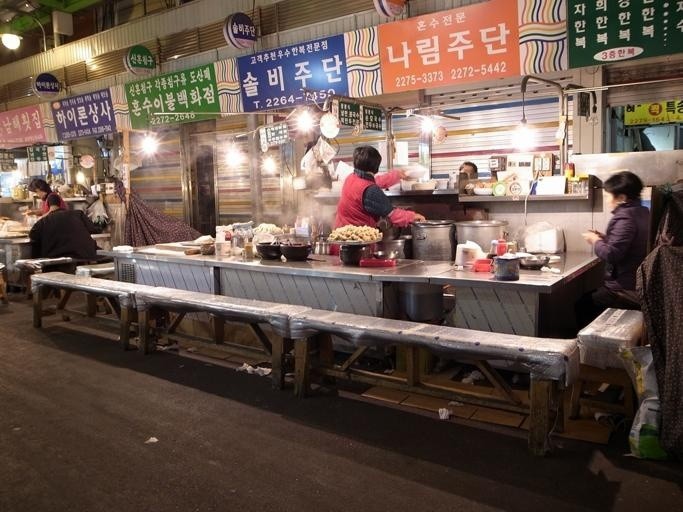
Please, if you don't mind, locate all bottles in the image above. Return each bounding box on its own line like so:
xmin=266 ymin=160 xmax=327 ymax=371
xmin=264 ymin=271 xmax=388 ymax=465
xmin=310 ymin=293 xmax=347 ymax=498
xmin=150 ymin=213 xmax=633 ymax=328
xmin=496 ymin=239 xmax=507 ymax=255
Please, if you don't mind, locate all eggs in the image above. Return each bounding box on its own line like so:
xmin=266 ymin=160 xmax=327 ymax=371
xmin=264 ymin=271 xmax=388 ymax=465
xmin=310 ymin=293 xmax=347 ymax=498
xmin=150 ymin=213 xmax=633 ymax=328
xmin=479 ymin=183 xmax=489 ymax=188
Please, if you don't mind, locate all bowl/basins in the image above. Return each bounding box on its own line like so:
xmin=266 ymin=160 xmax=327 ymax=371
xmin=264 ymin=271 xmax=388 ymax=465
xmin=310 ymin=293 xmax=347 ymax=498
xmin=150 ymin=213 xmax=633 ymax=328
xmin=279 ymin=243 xmax=312 ymax=261
xmin=414 ymin=182 xmax=436 ymax=190
xmin=521 ymin=257 xmax=550 ymax=270
xmin=406 ymin=170 xmax=425 ymax=179
xmin=256 ymin=241 xmax=283 ymax=260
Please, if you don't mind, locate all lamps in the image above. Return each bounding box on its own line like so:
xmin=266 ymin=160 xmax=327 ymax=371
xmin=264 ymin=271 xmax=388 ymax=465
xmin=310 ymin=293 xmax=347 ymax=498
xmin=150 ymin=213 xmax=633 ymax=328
xmin=390 ymin=109 xmax=434 ymax=170
xmin=296 ymin=89 xmax=390 ymax=171
xmin=141 ymin=112 xmax=290 ymax=154
xmin=564 ymin=84 xmax=598 ymax=163
xmin=225 ymin=129 xmax=259 ymax=167
xmin=0 ymin=12 xmax=47 ymax=52
xmin=517 ymin=75 xmax=564 ymax=176
xmin=76 ymin=157 xmax=111 ymax=183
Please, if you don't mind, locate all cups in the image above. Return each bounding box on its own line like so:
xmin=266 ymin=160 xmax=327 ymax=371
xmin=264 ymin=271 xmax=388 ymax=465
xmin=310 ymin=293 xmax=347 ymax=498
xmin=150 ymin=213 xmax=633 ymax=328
xmin=492 ymin=256 xmax=521 ymax=280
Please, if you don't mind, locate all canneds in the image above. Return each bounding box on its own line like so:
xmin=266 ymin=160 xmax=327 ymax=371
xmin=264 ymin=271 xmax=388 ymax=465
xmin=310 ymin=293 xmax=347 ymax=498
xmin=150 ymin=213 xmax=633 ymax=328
xmin=241 ymin=241 xmax=253 ymax=262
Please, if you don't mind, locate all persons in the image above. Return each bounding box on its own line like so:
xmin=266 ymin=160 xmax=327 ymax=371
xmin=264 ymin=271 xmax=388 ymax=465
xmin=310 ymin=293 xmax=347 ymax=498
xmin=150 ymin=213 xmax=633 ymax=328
xmin=334 ymin=145 xmax=426 ymax=231
xmin=574 ymin=170 xmax=650 ymax=330
xmin=28 ymin=206 xmax=103 ymax=299
xmin=21 ymin=178 xmax=68 ymax=216
xmin=459 ymin=161 xmax=479 ymax=179
